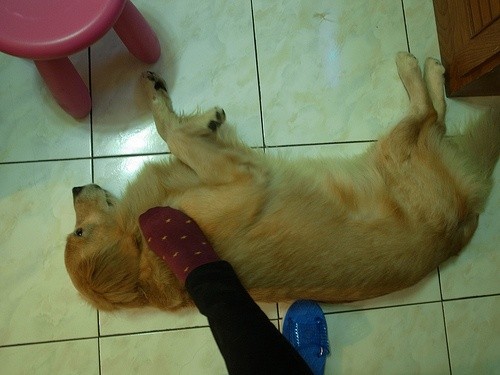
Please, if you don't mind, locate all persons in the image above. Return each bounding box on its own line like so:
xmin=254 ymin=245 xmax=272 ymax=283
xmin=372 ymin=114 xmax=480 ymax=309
xmin=138 ymin=206 xmax=316 ymax=375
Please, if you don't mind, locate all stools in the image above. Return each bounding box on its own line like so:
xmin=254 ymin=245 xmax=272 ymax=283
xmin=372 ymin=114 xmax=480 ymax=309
xmin=0 ymin=0 xmax=163 ymax=122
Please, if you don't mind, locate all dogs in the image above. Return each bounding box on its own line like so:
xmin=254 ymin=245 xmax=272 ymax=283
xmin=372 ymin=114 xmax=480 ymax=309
xmin=63 ymin=52 xmax=497 ymax=315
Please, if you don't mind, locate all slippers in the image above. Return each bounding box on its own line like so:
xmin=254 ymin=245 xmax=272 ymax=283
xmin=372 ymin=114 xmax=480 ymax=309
xmin=281 ymin=300 xmax=332 ymax=375
